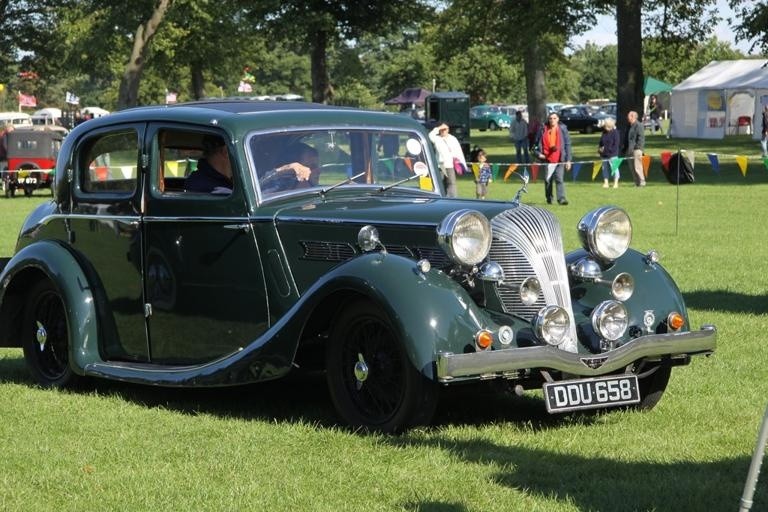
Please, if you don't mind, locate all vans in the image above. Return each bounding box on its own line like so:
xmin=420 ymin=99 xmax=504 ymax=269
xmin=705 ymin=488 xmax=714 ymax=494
xmin=0 ymin=111 xmax=34 ymax=129
xmin=31 ymin=107 xmax=69 ymax=128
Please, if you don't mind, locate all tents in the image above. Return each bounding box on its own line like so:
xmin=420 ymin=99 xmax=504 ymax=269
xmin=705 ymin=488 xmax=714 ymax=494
xmin=643 ymin=76 xmax=672 ymax=120
xmin=670 ymin=59 xmax=768 ymax=140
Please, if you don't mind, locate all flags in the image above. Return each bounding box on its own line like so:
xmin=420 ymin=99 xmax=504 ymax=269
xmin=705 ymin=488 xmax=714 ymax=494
xmin=238 ymin=81 xmax=252 ymax=92
xmin=66 ymin=91 xmax=80 ymax=105
xmin=20 ymin=94 xmax=37 ymax=108
xmin=167 ymin=93 xmax=177 ymax=102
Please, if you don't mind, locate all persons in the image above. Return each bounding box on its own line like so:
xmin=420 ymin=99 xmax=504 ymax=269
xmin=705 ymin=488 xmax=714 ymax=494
xmin=598 ymin=118 xmax=620 ymax=189
xmin=0 ymin=125 xmax=15 ymax=190
xmin=474 ymin=153 xmax=493 ymax=201
xmin=279 ymin=143 xmax=321 ymax=189
xmin=428 ymin=121 xmax=469 ymax=199
xmin=649 ymin=94 xmax=665 ymax=136
xmin=510 ymin=111 xmax=530 ymax=163
xmin=761 ymin=105 xmax=768 ymax=157
xmin=533 ymin=112 xmax=572 ymax=205
xmin=186 ymin=134 xmax=312 ymax=193
xmin=378 ymin=134 xmax=400 ymax=178
xmin=576 ymin=98 xmax=589 ymax=133
xmin=622 ymin=110 xmax=647 ymax=188
xmin=74 ymin=108 xmax=110 ymax=169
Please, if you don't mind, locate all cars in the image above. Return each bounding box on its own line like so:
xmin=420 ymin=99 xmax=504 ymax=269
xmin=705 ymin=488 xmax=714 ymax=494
xmin=400 ymin=107 xmax=418 ymax=120
xmin=80 ymin=105 xmax=111 ymax=118
xmin=0 ymin=130 xmax=63 ymax=198
xmin=128 ymin=217 xmax=280 ymax=317
xmin=1 ymin=100 xmax=718 ymax=434
xmin=469 ymin=102 xmax=617 ymax=135
